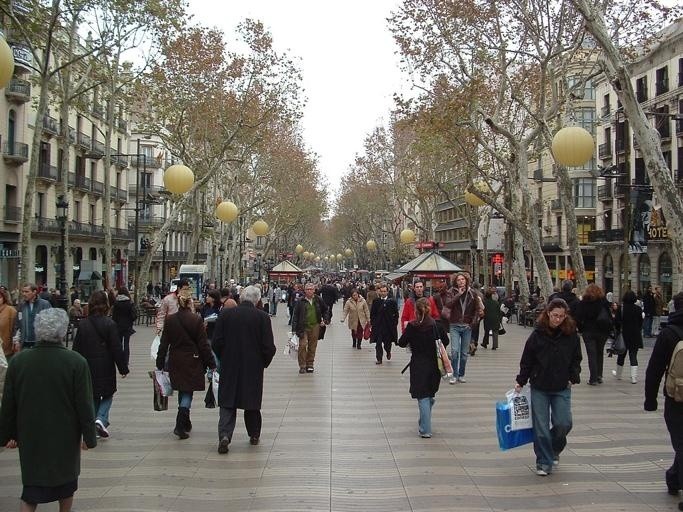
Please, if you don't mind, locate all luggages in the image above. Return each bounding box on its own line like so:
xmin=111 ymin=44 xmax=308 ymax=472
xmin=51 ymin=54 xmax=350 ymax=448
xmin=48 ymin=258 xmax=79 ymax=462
xmin=264 ymin=299 xmax=269 ymax=314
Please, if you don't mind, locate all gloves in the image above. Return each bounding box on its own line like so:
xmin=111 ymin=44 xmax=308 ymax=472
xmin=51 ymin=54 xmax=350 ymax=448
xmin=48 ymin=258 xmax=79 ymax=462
xmin=643 ymin=398 xmax=658 ymax=410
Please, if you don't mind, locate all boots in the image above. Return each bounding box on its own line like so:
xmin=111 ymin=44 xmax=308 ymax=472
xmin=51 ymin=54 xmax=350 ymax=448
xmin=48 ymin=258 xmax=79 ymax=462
xmin=630 ymin=366 xmax=639 ymax=383
xmin=612 ymin=365 xmax=623 ymax=379
xmin=174 ymin=408 xmax=188 ymax=439
xmin=470 ymin=343 xmax=476 ymax=355
xmin=178 ymin=407 xmax=192 ymax=432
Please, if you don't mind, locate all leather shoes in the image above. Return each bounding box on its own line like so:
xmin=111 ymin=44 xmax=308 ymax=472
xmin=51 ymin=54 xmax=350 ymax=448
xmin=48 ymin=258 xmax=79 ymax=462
xmin=491 ymin=348 xmax=496 ymax=350
xmin=480 ymin=343 xmax=487 ymax=348
xmin=387 ymin=353 xmax=392 ymax=359
xmin=375 ymin=359 xmax=382 ymax=364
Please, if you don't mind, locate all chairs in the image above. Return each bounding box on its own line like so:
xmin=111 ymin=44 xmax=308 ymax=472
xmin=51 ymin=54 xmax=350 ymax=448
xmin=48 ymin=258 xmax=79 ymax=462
xmin=507 ymin=307 xmax=545 ymax=328
xmin=134 ymin=306 xmax=159 ymax=327
xmin=64 ymin=314 xmax=88 ymax=347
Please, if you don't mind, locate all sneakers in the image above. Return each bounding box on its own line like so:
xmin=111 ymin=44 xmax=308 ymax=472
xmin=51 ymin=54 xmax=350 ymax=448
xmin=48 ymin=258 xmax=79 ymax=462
xmin=219 ymin=437 xmax=229 ymax=453
xmin=357 ymin=344 xmax=361 ymax=348
xmin=667 ymin=487 xmax=679 ymax=494
xmin=299 ymin=369 xmax=305 ymax=373
xmin=207 ymin=369 xmax=212 ymax=380
xmin=596 ymin=377 xmax=602 ymax=383
xmin=459 ymin=377 xmax=466 ymax=382
xmin=553 ymin=457 xmax=559 ymax=465
xmin=307 ymin=366 xmax=314 ymax=372
xmin=449 ymin=377 xmax=458 ymax=382
xmin=418 ymin=433 xmax=431 ymax=438
xmin=95 ymin=419 xmax=109 ymax=438
xmin=353 ymin=343 xmax=356 ymax=347
xmin=587 ymin=379 xmax=595 ymax=384
xmin=250 ymin=437 xmax=258 ymax=445
xmin=536 ymin=469 xmax=547 ymax=476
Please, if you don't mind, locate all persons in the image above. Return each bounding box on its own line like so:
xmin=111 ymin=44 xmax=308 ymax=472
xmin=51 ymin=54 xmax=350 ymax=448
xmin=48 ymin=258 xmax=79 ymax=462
xmin=287 ymin=272 xmax=400 ymax=373
xmin=513 ymin=277 xmax=682 ymax=495
xmin=398 ymin=270 xmax=501 ymax=436
xmin=146 ymin=276 xmax=288 ymax=454
xmin=0 ymin=269 xmax=137 ymax=512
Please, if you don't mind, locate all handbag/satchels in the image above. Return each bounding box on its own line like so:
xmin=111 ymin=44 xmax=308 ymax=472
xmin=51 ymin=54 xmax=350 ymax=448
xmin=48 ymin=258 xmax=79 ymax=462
xmin=496 ymin=400 xmax=534 ymax=451
xmin=499 ymin=328 xmax=506 ymax=334
xmin=292 ymin=334 xmax=299 ymax=350
xmin=284 ymin=344 xmax=289 ymax=355
xmin=156 ymin=372 xmax=172 ymax=397
xmin=150 ymin=335 xmax=161 ymax=362
xmin=505 ymin=382 xmax=533 ymax=431
xmin=318 ymin=326 xmax=326 ymax=339
xmin=364 ymin=321 xmax=371 ymax=340
xmin=598 ymin=308 xmax=612 ymax=331
xmin=606 ymin=333 xmax=626 ymax=357
xmin=435 ymin=339 xmax=446 ymax=376
xmin=256 ymin=301 xmax=262 ymax=310
xmin=438 ymin=339 xmax=452 ymax=373
xmin=149 ymin=371 xmax=168 ymax=411
xmin=288 ymin=332 xmax=298 ymax=360
xmin=212 ymin=373 xmax=219 ymax=406
xmin=204 ymin=382 xmax=215 ymax=408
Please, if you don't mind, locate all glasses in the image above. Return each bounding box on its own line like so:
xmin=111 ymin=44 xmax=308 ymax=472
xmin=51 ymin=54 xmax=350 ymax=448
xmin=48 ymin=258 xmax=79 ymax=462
xmin=551 ymin=314 xmax=564 ymax=320
xmin=306 ymin=289 xmax=313 ymax=290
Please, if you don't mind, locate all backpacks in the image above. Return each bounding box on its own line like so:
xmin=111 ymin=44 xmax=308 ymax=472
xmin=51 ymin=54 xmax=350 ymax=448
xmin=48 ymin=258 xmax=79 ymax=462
xmin=666 ymin=325 xmax=683 ymax=402
